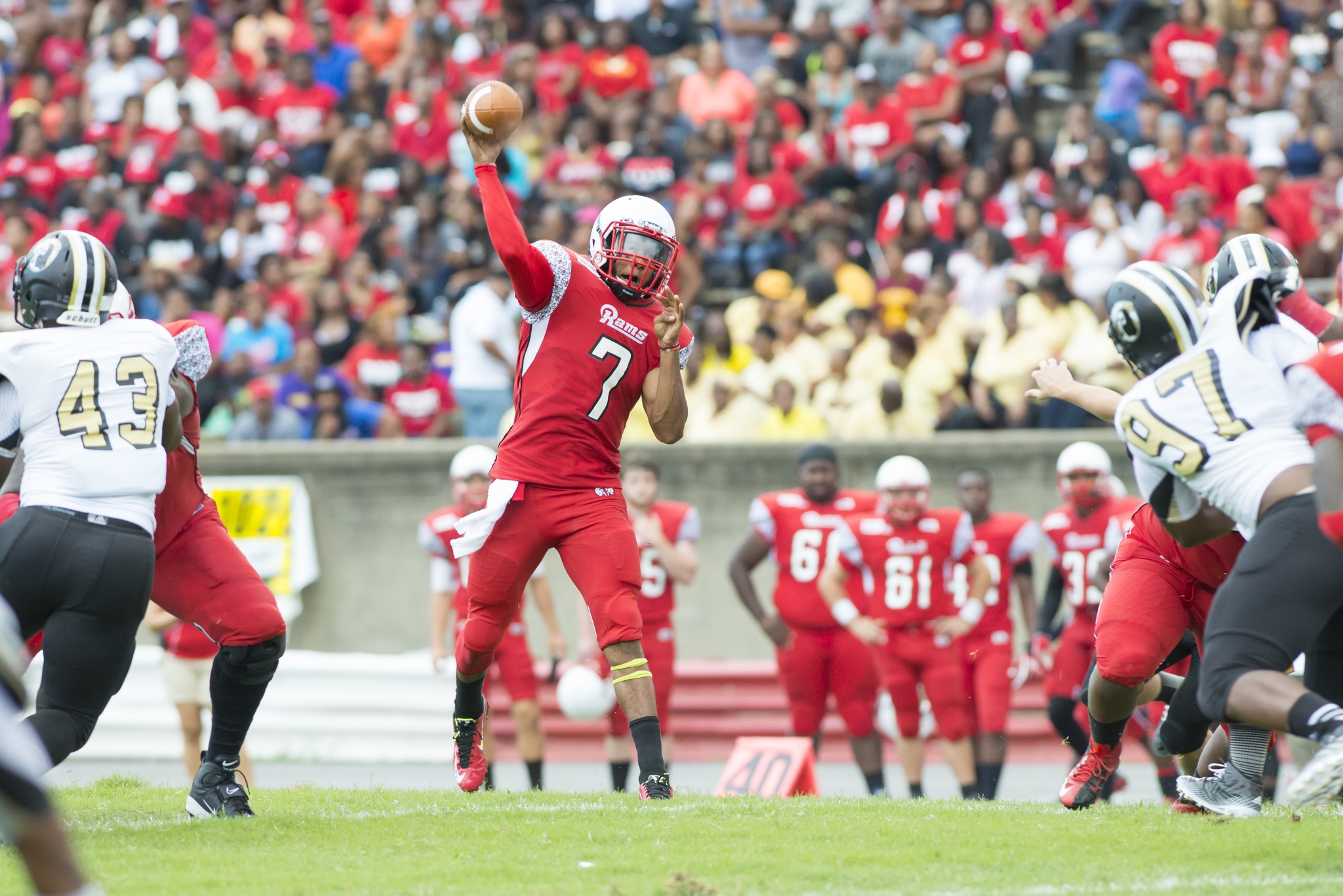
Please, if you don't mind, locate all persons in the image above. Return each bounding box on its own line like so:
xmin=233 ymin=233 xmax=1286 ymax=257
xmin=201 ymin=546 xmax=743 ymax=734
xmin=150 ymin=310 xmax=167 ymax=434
xmin=0 ymin=271 xmax=302 ymax=827
xmin=0 ymin=0 xmax=1343 ymax=450
xmin=1075 ymin=467 xmax=1204 ymax=802
xmin=147 ymin=594 xmax=255 ymax=794
xmin=447 ymin=107 xmax=697 ymax=816
xmin=1055 ymin=468 xmax=1264 ymax=816
xmin=1283 ymin=343 xmax=1342 ymax=545
xmin=0 ymin=598 xmax=100 ymax=896
xmin=925 ymin=464 xmax=1041 ymax=806
xmin=415 ymin=440 xmax=558 ymax=793
xmin=584 ymin=451 xmax=702 ymax=802
xmin=1103 ymin=257 xmax=1342 ymax=828
xmin=726 ymin=440 xmax=890 ymax=809
xmin=814 ymin=450 xmax=1000 ymax=813
xmin=2 ymin=222 xmax=178 ymax=785
xmin=1020 ymin=439 xmax=1180 ymax=798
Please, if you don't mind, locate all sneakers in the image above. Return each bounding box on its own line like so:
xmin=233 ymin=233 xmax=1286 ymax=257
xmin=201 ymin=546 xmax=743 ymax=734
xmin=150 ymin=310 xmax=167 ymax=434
xmin=453 ymin=694 xmax=490 ymax=792
xmin=185 ymin=750 xmax=255 ymax=818
xmin=1171 ymin=792 xmax=1209 ymax=815
xmin=1175 ymin=760 xmax=1266 ymax=817
xmin=639 ymin=772 xmax=673 ymax=800
xmin=1285 ymin=719 xmax=1343 ymax=810
xmin=1059 ymin=735 xmax=1123 ymax=811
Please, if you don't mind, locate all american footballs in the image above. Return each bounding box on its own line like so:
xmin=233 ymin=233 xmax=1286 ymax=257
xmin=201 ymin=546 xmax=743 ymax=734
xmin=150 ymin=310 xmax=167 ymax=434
xmin=463 ymin=80 xmax=522 ymax=145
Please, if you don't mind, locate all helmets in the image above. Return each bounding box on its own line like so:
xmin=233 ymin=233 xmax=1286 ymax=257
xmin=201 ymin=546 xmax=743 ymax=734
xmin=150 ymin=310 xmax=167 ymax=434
xmin=1105 ymin=259 xmax=1209 ymax=382
xmin=12 ymin=230 xmax=119 ymax=329
xmin=876 ymin=455 xmax=931 ymax=499
xmin=1207 ymin=233 xmax=1300 ymax=306
xmin=556 ymin=666 xmax=617 ymax=722
xmin=449 ymin=444 xmax=497 ymax=487
xmin=1057 ymin=440 xmax=1111 ymax=488
xmin=588 ymin=194 xmax=677 ymax=280
